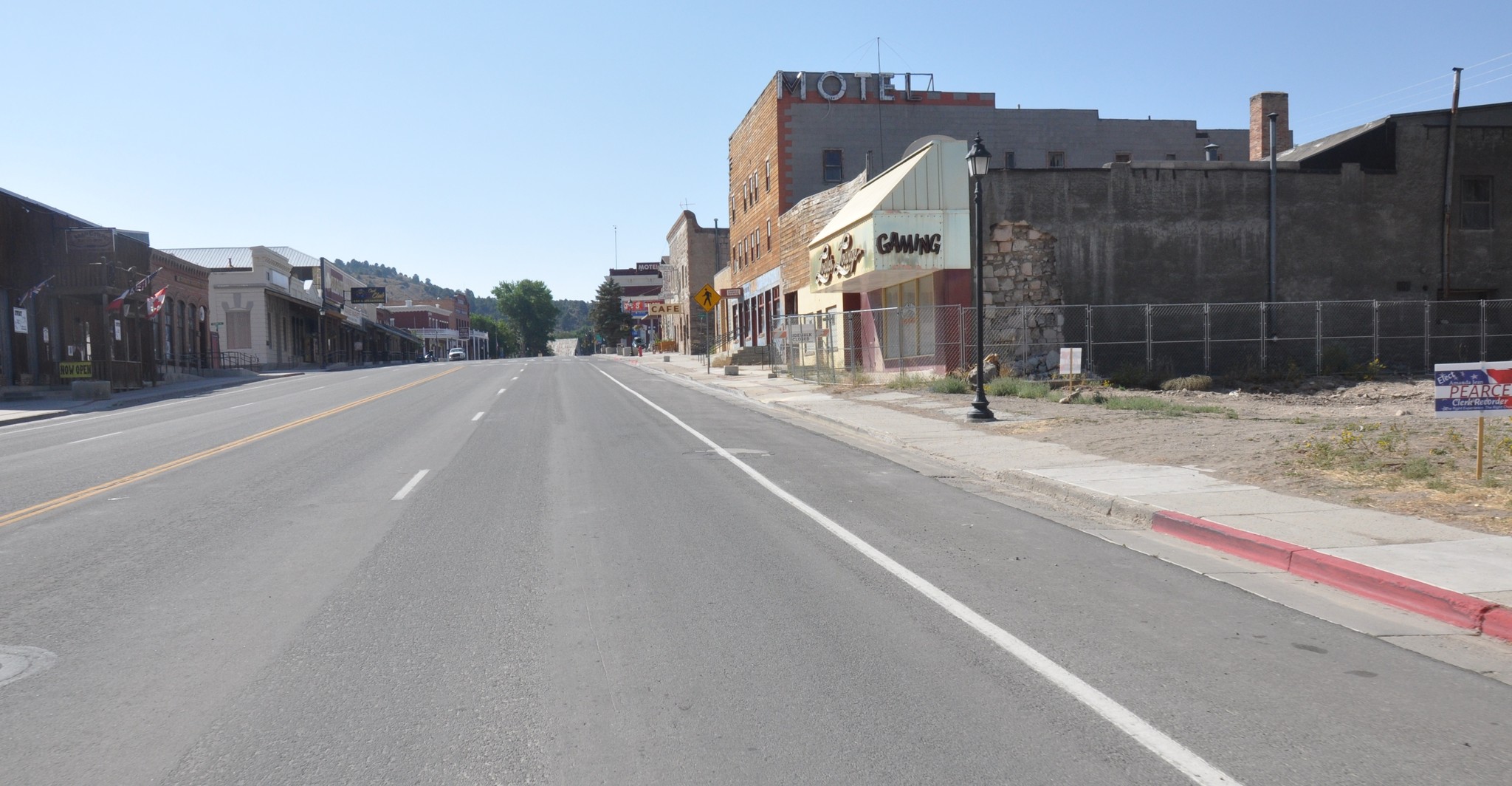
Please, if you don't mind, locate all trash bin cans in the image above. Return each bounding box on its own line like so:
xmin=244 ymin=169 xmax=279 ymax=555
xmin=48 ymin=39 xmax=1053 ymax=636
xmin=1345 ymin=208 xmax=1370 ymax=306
xmin=601 ymin=345 xmax=606 ymax=354
xmin=382 ymin=351 xmax=389 ymax=361
xmin=616 ymin=344 xmax=624 ymax=355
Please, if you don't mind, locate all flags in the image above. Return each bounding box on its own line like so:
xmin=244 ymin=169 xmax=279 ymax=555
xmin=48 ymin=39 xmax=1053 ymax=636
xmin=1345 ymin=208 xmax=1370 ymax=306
xmin=18 ymin=275 xmax=55 ymax=307
xmin=107 ymin=266 xmax=170 ymax=320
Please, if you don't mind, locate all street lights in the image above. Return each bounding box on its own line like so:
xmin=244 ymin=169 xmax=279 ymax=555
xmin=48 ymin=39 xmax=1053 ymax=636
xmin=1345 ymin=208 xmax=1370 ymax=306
xmin=434 ymin=330 xmax=439 ymax=362
xmin=319 ymin=306 xmax=327 ymax=369
xmin=964 ymin=129 xmax=997 ymax=424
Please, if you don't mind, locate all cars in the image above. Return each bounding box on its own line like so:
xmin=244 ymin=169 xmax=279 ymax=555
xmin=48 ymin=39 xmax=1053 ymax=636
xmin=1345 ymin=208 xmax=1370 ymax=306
xmin=631 ymin=337 xmax=648 ymax=349
xmin=449 ymin=347 xmax=466 ymax=361
